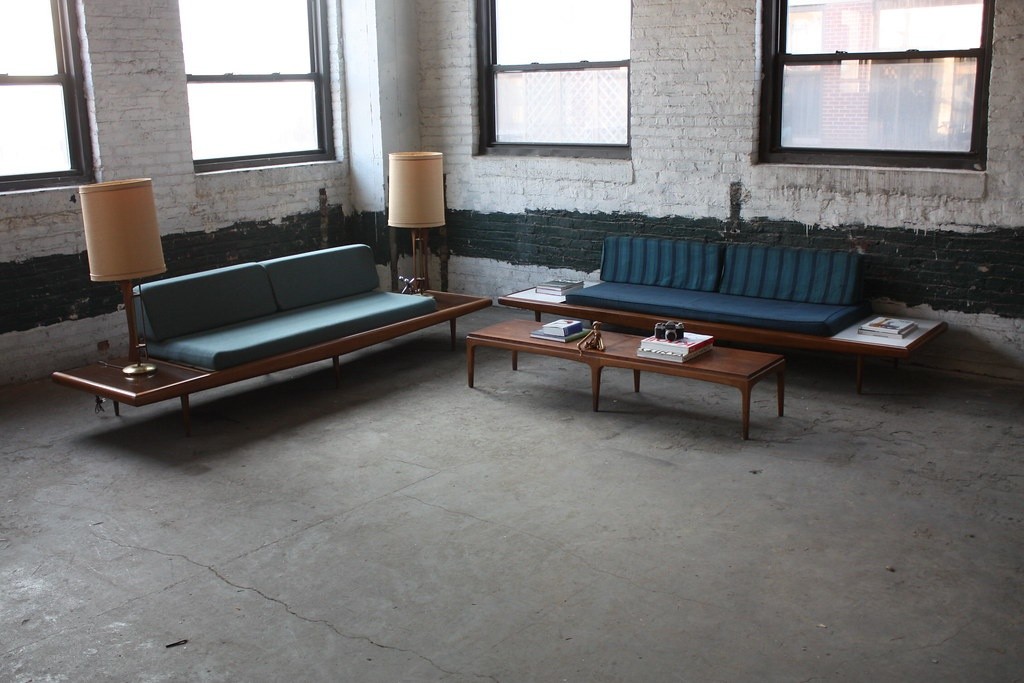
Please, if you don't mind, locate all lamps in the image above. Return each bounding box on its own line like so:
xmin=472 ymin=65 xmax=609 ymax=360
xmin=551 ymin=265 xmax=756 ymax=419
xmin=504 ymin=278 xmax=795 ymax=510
xmin=387 ymin=150 xmax=447 ymax=294
xmin=74 ymin=176 xmax=170 ymax=379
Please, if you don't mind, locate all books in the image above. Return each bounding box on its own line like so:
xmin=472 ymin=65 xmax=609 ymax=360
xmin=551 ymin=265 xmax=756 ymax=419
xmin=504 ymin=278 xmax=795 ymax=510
xmin=535 ymin=279 xmax=585 ymax=296
xmin=637 ymin=332 xmax=715 ymax=363
xmin=529 ymin=318 xmax=591 ymax=342
xmin=858 ymin=316 xmax=919 ymax=339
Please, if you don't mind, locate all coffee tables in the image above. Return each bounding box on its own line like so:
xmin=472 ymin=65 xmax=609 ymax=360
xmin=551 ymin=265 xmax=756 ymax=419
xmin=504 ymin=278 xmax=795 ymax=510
xmin=466 ymin=318 xmax=787 ymax=439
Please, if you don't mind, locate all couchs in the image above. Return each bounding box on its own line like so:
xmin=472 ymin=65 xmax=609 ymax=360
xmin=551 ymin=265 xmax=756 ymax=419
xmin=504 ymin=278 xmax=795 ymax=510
xmin=498 ymin=232 xmax=947 ymax=397
xmin=53 ymin=243 xmax=494 ymax=436
